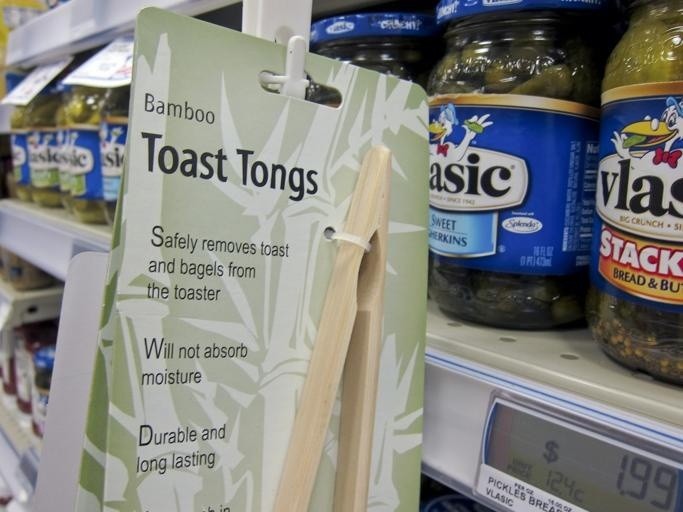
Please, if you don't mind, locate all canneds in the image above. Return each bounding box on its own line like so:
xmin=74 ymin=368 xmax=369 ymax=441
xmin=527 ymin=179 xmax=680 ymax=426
xmin=6 ymin=72 xmax=130 ymax=225
xmin=585 ymin=2 xmax=683 ymax=385
xmin=306 ymin=11 xmax=442 ymax=108
xmin=427 ymin=0 xmax=621 ymax=332
xmin=0 ymin=325 xmax=56 ymax=438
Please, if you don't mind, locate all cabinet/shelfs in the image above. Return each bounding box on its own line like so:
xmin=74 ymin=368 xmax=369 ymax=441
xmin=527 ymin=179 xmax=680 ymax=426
xmin=4 ymin=1 xmax=681 ymax=511
